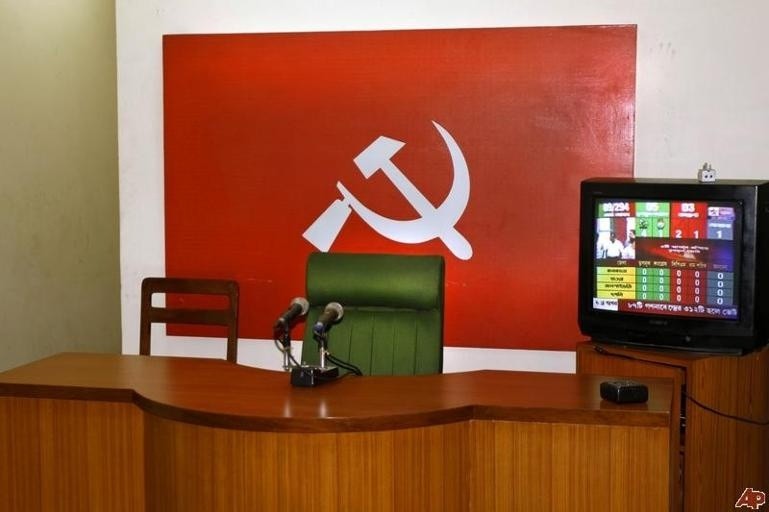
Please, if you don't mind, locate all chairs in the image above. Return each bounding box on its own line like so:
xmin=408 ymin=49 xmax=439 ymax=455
xmin=301 ymin=250 xmax=446 ymax=375
xmin=139 ymin=275 xmax=239 ymax=364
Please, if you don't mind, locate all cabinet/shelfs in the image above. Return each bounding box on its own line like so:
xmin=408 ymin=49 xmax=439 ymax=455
xmin=571 ymin=341 xmax=768 ymax=512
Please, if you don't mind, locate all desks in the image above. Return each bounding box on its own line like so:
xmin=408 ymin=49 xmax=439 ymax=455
xmin=0 ymin=353 xmax=679 ymax=512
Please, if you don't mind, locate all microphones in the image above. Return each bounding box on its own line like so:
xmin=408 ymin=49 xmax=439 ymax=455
xmin=313 ymin=302 xmax=344 ymax=331
xmin=273 ymin=296 xmax=310 ymax=331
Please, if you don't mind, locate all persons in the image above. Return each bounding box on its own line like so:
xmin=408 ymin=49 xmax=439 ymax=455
xmin=595 ymin=229 xmax=636 ymax=259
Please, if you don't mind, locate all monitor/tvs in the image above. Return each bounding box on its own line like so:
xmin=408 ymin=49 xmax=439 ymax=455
xmin=578 ymin=177 xmax=769 ymax=358
